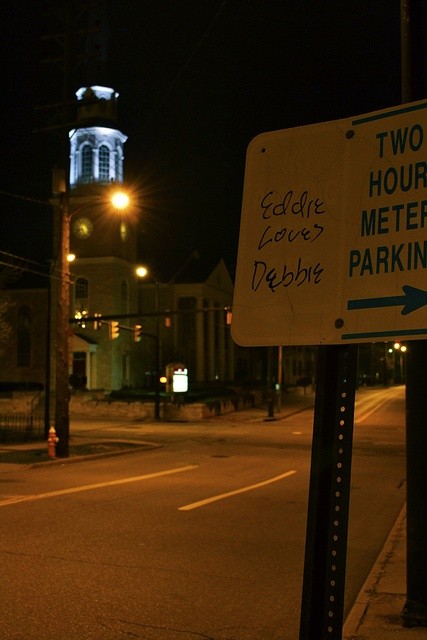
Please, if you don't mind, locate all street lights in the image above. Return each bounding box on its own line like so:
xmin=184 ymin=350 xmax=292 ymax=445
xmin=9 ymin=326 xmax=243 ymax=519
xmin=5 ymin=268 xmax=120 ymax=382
xmin=135 ymin=267 xmax=159 ymax=419
xmin=48 ymin=191 xmax=128 ymax=458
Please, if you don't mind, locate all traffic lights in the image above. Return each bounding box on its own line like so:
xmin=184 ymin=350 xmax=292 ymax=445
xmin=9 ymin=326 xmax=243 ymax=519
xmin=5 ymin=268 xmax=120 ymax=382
xmin=109 ymin=320 xmax=119 ymax=340
xmin=133 ymin=324 xmax=142 ymax=343
xmin=75 ymin=311 xmax=82 ymax=325
xmin=81 ymin=310 xmax=88 ymax=329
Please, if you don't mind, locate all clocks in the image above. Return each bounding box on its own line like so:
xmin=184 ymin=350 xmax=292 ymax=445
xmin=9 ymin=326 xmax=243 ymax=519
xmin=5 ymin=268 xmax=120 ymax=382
xmin=72 ymin=217 xmax=93 ymax=240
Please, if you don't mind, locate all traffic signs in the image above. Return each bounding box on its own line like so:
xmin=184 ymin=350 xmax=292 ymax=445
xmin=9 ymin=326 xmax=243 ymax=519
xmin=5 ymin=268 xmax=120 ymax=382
xmin=230 ymin=98 xmax=426 ymax=347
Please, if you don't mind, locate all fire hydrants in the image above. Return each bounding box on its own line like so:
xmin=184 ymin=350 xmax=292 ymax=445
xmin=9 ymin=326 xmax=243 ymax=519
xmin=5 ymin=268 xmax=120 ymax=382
xmin=48 ymin=427 xmax=58 ymax=458
